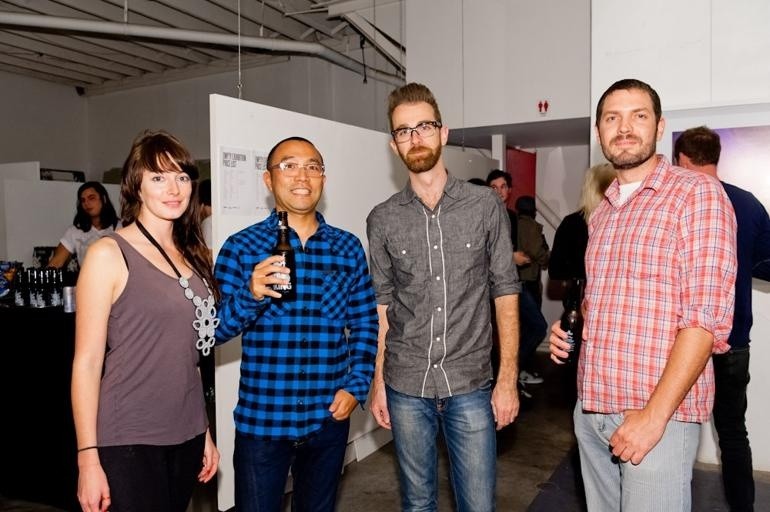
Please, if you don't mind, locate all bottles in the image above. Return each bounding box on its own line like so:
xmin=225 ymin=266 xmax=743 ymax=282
xmin=560 ymin=279 xmax=583 ymax=365
xmin=273 ymin=210 xmax=297 ymax=300
xmin=11 ymin=263 xmax=76 ymax=313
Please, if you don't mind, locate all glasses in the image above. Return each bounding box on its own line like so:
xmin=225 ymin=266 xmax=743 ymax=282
xmin=391 ymin=121 xmax=443 ymax=143
xmin=268 ymin=161 xmax=325 ymax=178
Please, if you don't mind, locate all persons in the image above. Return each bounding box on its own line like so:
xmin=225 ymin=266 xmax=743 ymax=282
xmin=545 ymin=205 xmax=590 ymax=314
xmin=365 ymin=83 xmax=521 ymax=511
xmin=673 ymin=126 xmax=770 ymax=512
xmin=548 ymin=79 xmax=740 ymax=512
xmin=71 ymin=129 xmax=219 ymax=512
xmin=213 ymin=138 xmax=378 ymax=512
xmin=45 ymin=181 xmax=120 ymax=269
xmin=198 ymin=178 xmax=214 ymax=252
xmin=468 ymin=167 xmax=550 ymax=383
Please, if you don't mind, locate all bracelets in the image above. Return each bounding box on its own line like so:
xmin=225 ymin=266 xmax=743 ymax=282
xmin=76 ymin=446 xmax=100 ymax=452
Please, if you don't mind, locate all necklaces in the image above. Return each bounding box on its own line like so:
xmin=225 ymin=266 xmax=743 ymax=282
xmin=131 ymin=215 xmax=218 ymax=356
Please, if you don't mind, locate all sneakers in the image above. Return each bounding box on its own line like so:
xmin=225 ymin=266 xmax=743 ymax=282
xmin=520 ymin=369 xmax=546 ymax=384
